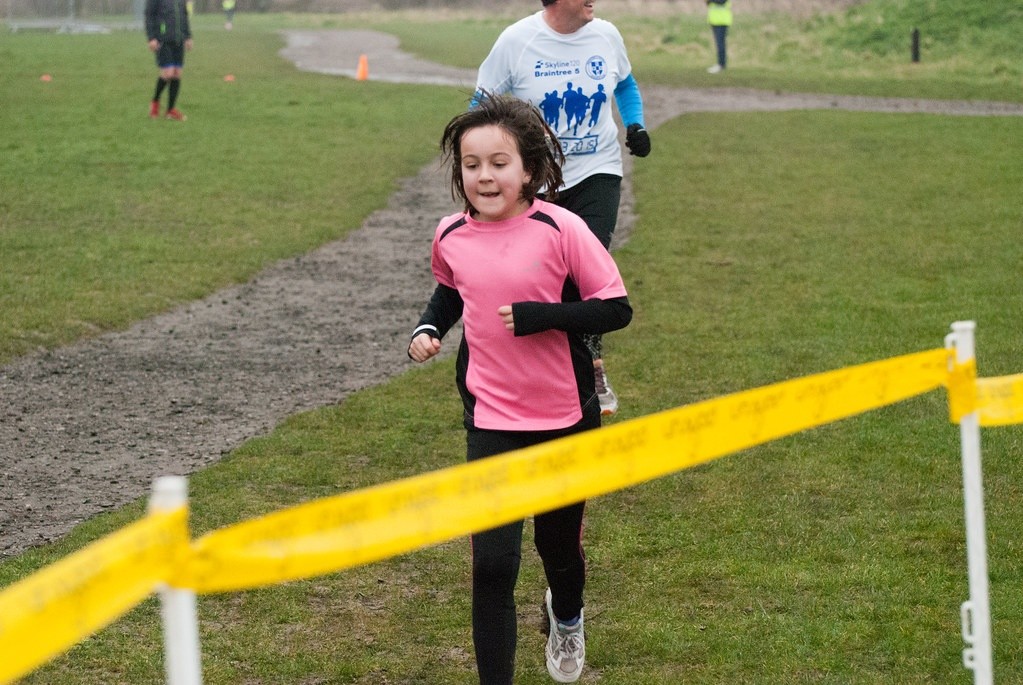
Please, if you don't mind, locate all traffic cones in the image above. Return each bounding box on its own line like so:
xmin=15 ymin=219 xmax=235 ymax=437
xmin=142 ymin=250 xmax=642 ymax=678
xmin=354 ymin=54 xmax=370 ymax=81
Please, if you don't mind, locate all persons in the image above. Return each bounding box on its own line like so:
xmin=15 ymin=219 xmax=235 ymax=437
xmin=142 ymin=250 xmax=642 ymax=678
xmin=407 ymin=83 xmax=635 ymax=685
xmin=471 ymin=1 xmax=651 ymax=417
xmin=703 ymin=1 xmax=732 ymax=76
xmin=221 ymin=0 xmax=235 ymax=28
xmin=146 ymin=0 xmax=192 ymax=121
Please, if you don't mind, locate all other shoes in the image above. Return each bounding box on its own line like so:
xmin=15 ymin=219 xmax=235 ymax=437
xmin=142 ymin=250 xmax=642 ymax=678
xmin=151 ymin=101 xmax=161 ymax=116
xmin=165 ymin=109 xmax=187 ymax=121
xmin=710 ymin=66 xmax=724 ymax=74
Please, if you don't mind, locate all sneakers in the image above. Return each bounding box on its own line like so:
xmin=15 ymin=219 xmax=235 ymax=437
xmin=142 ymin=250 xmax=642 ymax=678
xmin=543 ymin=586 xmax=585 ymax=682
xmin=595 ymin=359 xmax=629 ymax=414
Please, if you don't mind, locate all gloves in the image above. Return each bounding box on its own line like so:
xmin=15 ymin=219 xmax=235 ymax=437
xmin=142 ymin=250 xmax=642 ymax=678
xmin=624 ymin=124 xmax=654 ymax=160
